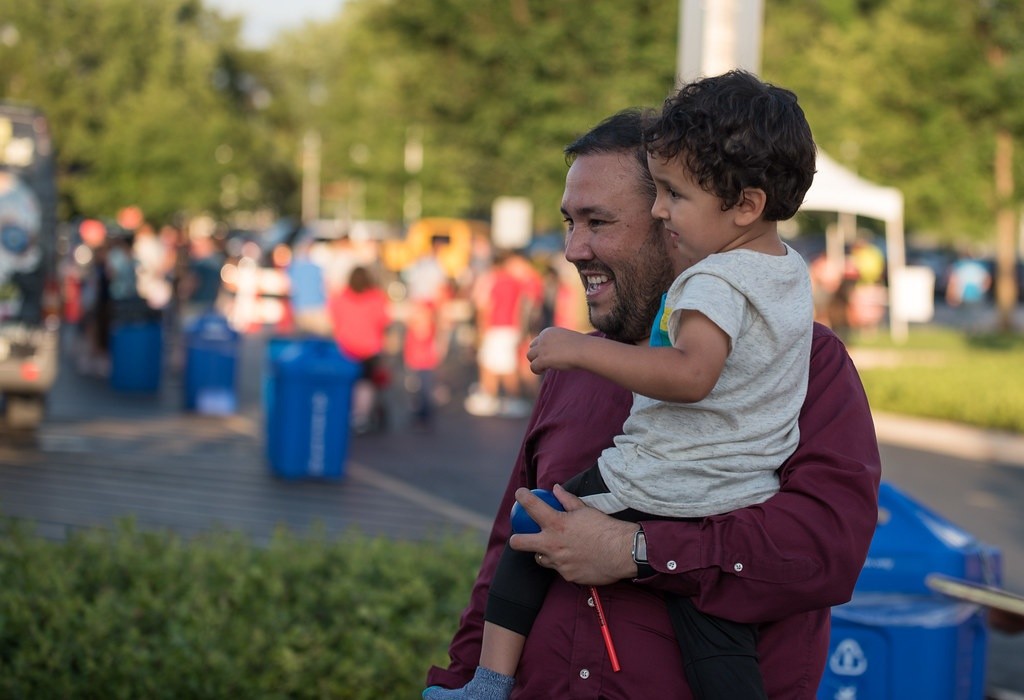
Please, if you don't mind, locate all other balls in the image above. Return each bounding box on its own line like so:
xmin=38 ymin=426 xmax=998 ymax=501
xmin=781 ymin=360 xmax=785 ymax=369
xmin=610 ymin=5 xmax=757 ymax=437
xmin=511 ymin=488 xmax=564 ymax=536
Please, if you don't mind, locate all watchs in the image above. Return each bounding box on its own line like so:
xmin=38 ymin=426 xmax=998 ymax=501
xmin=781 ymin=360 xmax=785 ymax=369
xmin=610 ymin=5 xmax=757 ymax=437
xmin=632 ymin=526 xmax=656 ymax=580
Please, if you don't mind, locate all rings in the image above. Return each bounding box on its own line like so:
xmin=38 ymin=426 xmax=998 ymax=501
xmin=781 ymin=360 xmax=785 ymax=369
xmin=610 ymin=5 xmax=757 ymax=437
xmin=539 ymin=554 xmax=542 ymax=563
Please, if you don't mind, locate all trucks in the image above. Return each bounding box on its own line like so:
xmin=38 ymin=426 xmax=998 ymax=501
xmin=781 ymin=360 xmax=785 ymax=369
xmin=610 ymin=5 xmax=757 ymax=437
xmin=0 ymin=98 xmax=57 ymax=452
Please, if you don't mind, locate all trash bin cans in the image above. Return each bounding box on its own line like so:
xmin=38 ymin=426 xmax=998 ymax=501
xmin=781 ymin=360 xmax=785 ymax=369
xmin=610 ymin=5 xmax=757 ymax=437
xmin=181 ymin=316 xmax=243 ymax=413
xmin=817 ymin=481 xmax=1005 ymax=700
xmin=107 ymin=301 xmax=167 ymax=395
xmin=260 ymin=334 xmax=363 ymax=483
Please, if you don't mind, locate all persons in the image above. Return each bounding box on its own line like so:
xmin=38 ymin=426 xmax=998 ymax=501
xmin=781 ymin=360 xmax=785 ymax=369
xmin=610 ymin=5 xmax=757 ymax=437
xmin=425 ymin=108 xmax=881 ymax=700
xmin=57 ymin=206 xmax=582 ymax=436
xmin=796 ymin=221 xmax=885 ymax=327
xmin=424 ymin=72 xmax=815 ymax=700
xmin=946 ymin=245 xmax=992 ymax=306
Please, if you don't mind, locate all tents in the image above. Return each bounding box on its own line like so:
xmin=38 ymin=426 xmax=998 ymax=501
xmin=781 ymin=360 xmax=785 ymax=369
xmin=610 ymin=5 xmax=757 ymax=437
xmin=800 ymin=147 xmax=907 ymax=340
